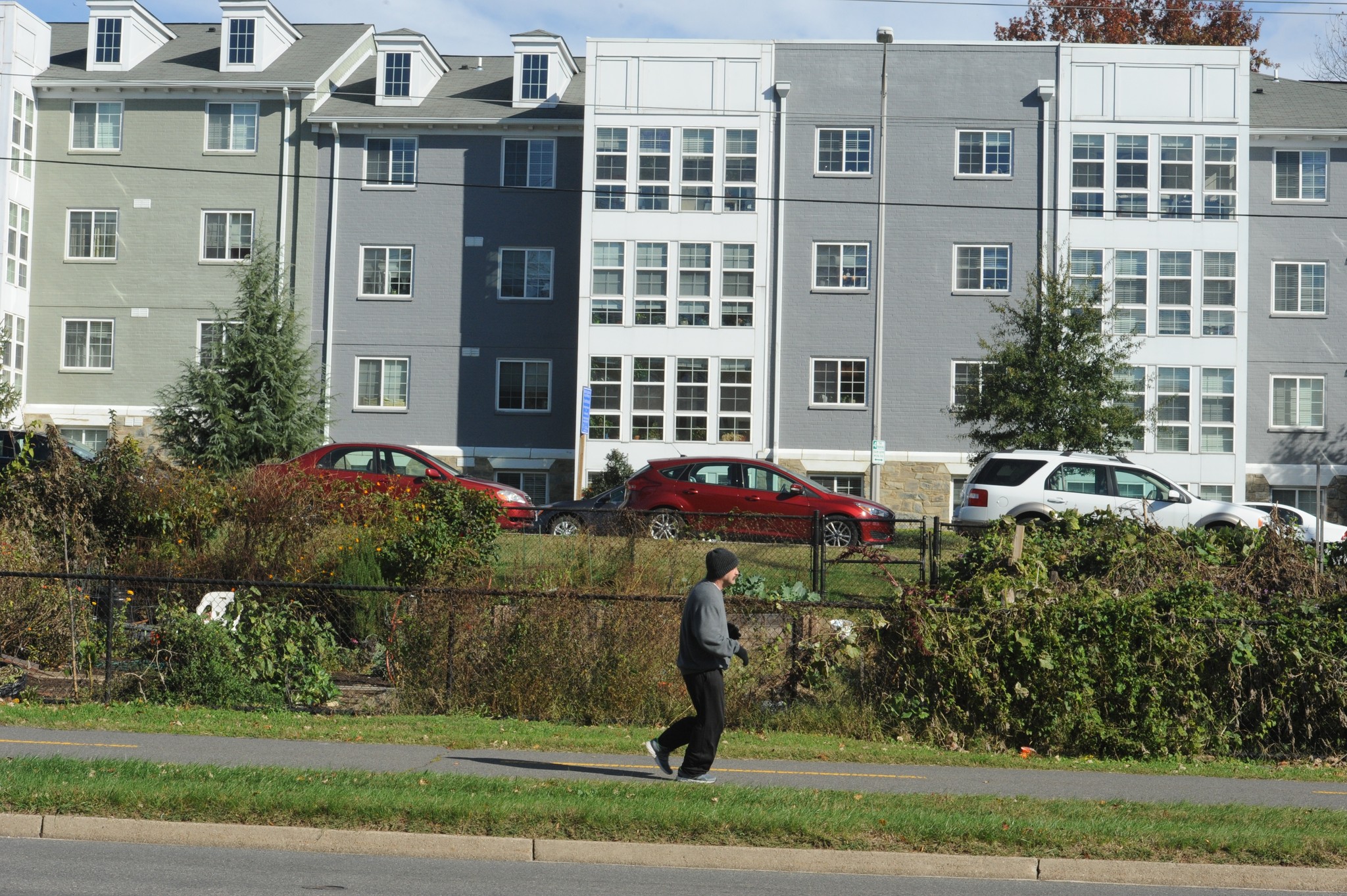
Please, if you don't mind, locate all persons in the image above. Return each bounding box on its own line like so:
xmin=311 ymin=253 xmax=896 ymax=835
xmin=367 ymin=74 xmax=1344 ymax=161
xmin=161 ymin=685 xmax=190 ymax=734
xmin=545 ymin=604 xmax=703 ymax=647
xmin=646 ymin=548 xmax=749 ymax=784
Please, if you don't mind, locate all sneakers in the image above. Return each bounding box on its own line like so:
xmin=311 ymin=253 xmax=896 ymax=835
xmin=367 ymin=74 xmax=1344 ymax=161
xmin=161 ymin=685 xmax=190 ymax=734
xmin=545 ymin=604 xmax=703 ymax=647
xmin=645 ymin=738 xmax=673 ymax=775
xmin=676 ymin=766 xmax=717 ymax=783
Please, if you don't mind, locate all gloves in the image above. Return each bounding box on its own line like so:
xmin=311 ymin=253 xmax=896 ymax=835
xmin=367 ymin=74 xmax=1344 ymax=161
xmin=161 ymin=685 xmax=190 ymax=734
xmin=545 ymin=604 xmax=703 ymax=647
xmin=735 ymin=645 xmax=749 ymax=667
xmin=726 ymin=623 xmax=742 ymax=640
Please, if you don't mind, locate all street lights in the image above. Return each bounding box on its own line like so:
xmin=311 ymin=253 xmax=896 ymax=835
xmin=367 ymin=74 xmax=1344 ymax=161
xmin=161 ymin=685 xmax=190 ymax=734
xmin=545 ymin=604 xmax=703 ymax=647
xmin=869 ymin=26 xmax=896 ymax=503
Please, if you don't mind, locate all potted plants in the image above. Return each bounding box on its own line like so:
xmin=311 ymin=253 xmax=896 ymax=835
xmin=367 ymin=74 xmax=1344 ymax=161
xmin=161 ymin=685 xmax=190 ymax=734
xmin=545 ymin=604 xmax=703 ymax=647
xmin=848 ymin=397 xmax=855 ymax=403
xmin=649 ymin=421 xmax=661 ymax=440
xmin=737 ymin=434 xmax=747 ymax=441
xmin=633 ymin=426 xmax=640 ymax=439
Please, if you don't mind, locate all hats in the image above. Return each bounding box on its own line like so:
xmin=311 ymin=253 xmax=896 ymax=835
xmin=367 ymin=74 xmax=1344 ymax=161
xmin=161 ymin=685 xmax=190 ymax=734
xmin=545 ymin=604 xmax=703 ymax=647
xmin=705 ymin=548 xmax=738 ymax=580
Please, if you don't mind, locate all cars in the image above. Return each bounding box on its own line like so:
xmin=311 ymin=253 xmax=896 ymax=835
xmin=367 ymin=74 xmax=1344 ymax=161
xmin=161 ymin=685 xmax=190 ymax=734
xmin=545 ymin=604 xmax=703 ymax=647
xmin=0 ymin=428 xmax=97 ymax=474
xmin=1234 ymin=501 xmax=1347 ymax=545
xmin=532 ymin=484 xmax=627 ymax=535
xmin=252 ymin=441 xmax=537 ymax=532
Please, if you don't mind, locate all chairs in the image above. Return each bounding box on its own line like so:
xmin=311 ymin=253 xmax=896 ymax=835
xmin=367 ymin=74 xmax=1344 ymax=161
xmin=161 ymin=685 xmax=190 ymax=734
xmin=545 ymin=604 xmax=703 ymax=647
xmin=196 ymin=592 xmax=244 ymax=630
xmin=381 ymin=459 xmax=387 ymax=470
xmin=366 ymin=459 xmax=372 ymax=471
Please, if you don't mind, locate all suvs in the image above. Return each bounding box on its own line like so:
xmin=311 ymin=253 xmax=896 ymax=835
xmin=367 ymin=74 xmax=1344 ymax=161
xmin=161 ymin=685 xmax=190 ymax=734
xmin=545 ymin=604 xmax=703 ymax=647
xmin=950 ymin=448 xmax=1271 ymax=537
xmin=617 ymin=456 xmax=897 ymax=549
